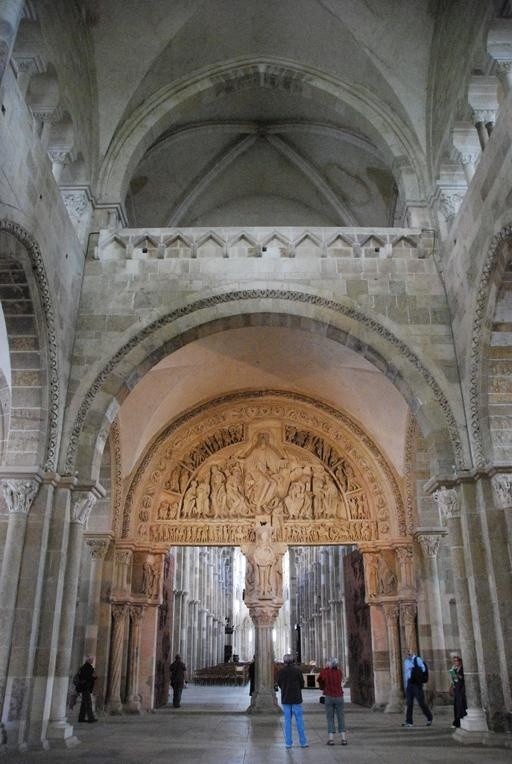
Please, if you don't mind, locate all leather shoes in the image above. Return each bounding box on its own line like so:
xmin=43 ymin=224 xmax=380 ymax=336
xmin=77 ymin=718 xmax=98 ymax=723
xmin=449 ymin=725 xmax=457 ymax=729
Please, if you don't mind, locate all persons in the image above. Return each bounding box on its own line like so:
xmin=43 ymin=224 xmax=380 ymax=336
xmin=76 ymin=654 xmax=99 ymax=723
xmin=276 ymin=652 xmax=309 ymax=748
xmin=170 ymin=653 xmax=186 ymax=707
xmin=147 ymin=553 xmax=162 ymax=600
xmin=140 ymin=563 xmax=151 ymax=593
xmin=273 ymin=663 xmax=278 ymax=691
xmin=248 ymin=654 xmax=255 ymax=697
xmin=372 ymin=553 xmax=395 ymax=594
xmin=447 ymin=656 xmax=466 ymax=729
xmin=399 ymin=647 xmax=433 ymax=728
xmin=159 ymin=418 xmax=370 ymax=540
xmin=319 ymin=657 xmax=347 ymax=745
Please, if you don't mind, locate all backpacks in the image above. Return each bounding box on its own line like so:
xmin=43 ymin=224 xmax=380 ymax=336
xmin=414 ymin=656 xmax=429 ymax=683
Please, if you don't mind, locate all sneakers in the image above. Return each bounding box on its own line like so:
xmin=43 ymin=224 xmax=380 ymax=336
xmin=301 ymin=743 xmax=309 ymax=748
xmin=400 ymin=721 xmax=413 ymax=727
xmin=285 ymin=744 xmax=292 ymax=749
xmin=424 ymin=719 xmax=433 ymax=726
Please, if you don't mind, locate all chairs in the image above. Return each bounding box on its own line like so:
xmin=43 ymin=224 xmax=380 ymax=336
xmin=190 ymin=656 xmax=328 ymax=690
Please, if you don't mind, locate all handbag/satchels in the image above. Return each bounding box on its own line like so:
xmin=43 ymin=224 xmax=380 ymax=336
xmin=316 ymin=670 xmax=326 ymax=690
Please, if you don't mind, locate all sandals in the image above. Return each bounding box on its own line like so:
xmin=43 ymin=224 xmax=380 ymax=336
xmin=327 ymin=740 xmax=335 ymax=745
xmin=341 ymin=739 xmax=347 ymax=745
